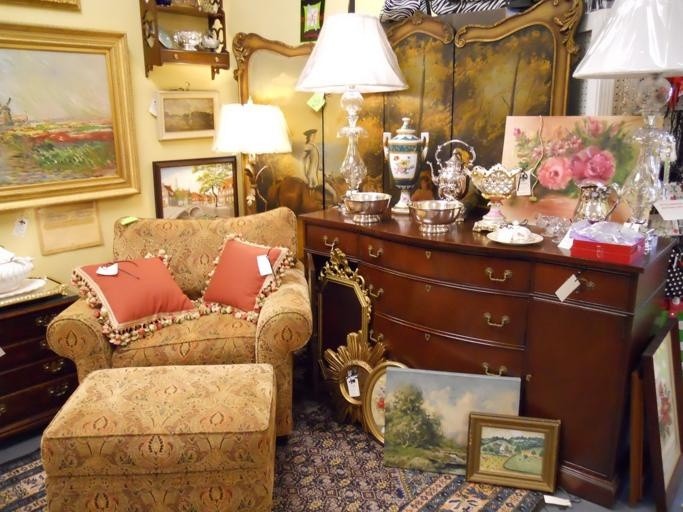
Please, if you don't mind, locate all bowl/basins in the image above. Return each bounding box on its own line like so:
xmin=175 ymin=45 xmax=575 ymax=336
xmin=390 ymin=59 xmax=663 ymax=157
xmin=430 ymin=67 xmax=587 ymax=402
xmin=340 ymin=192 xmax=392 ymax=224
xmin=461 ymin=163 xmax=522 ymax=233
xmin=407 ymin=200 xmax=461 ymax=234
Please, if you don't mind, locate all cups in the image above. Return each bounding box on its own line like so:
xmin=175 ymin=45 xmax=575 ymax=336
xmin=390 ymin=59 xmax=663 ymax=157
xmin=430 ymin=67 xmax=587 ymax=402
xmin=543 ymin=216 xmax=560 ymax=236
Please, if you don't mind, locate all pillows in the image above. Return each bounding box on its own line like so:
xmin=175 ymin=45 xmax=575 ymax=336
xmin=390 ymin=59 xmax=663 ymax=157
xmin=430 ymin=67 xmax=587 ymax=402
xmin=71 ymin=250 xmax=202 ymax=346
xmin=199 ymin=235 xmax=295 ymax=323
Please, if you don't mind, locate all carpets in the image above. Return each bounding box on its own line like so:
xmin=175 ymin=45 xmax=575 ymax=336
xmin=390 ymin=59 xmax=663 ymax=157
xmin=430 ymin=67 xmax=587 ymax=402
xmin=1 ymin=399 xmax=551 ymax=512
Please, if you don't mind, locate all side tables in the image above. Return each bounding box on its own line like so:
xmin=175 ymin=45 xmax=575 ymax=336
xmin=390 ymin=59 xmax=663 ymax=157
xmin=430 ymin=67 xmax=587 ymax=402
xmin=0 ymin=285 xmax=81 ymax=454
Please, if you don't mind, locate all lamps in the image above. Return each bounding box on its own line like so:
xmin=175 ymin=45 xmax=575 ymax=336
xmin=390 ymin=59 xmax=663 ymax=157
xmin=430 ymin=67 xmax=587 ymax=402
xmin=573 ymin=0 xmax=682 ymax=254
xmin=296 ymin=14 xmax=410 ymax=219
xmin=213 ymin=102 xmax=292 ymax=215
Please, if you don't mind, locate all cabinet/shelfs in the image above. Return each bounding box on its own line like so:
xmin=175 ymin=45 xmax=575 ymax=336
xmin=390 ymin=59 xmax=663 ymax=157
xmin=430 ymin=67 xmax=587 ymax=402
xmin=139 ymin=0 xmax=230 ymax=81
xmin=301 ymin=190 xmax=676 ymax=510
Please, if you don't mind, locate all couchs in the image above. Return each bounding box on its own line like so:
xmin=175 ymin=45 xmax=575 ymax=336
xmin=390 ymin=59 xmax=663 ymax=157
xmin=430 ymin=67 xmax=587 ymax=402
xmin=47 ymin=205 xmax=313 ymax=438
xmin=42 ymin=364 xmax=275 ymax=512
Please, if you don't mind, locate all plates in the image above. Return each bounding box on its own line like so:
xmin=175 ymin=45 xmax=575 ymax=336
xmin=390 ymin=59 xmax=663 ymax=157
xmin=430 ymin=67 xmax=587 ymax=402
xmin=486 ymin=231 xmax=545 ymax=246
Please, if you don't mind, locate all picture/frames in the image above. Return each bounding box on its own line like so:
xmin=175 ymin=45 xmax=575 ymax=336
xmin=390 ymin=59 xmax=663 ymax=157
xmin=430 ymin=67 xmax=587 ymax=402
xmin=33 ymin=201 xmax=104 ymax=257
xmin=317 ymin=261 xmax=367 ymax=383
xmin=361 ymin=361 xmax=408 ymax=446
xmin=151 ymin=157 xmax=238 ymax=220
xmin=298 ymin=1 xmax=324 ymax=42
xmin=154 ymin=90 xmax=218 ymax=142
xmin=642 ymin=317 xmax=683 ymax=511
xmin=0 ymin=66 xmax=141 ymax=210
xmin=466 ymin=411 xmax=561 ymax=494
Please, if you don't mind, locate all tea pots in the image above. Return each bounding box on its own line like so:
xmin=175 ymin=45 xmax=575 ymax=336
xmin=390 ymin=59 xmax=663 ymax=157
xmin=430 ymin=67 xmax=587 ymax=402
xmin=426 ymin=140 xmax=476 ymax=200
xmin=573 ymin=182 xmax=621 ymax=225
